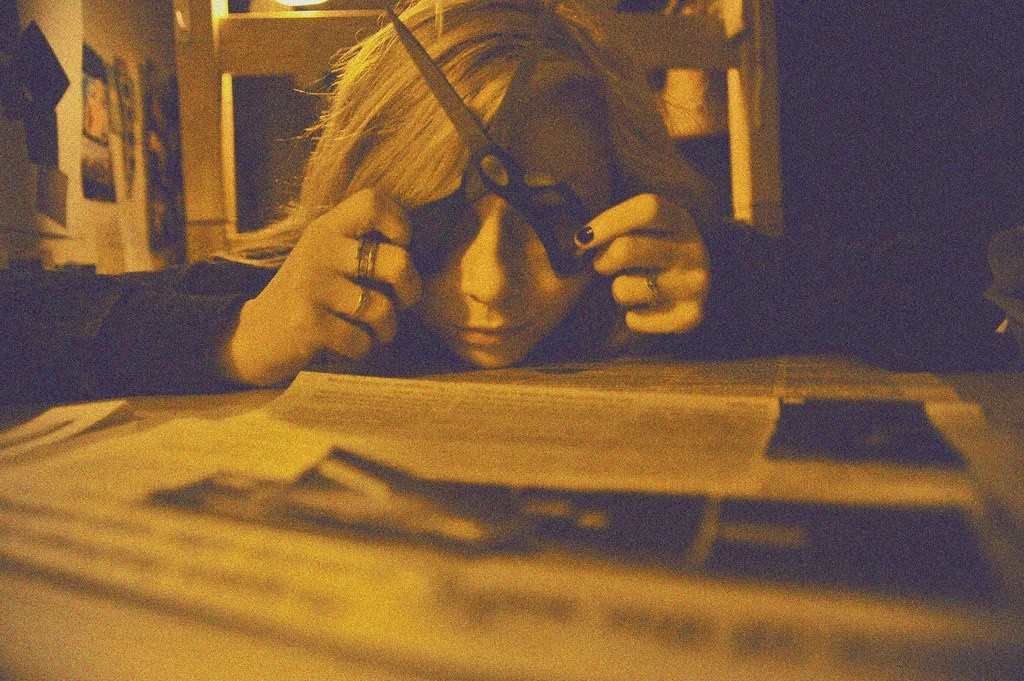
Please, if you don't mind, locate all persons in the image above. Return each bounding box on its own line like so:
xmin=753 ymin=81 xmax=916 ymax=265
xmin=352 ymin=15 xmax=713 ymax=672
xmin=0 ymin=0 xmax=1024 ymax=402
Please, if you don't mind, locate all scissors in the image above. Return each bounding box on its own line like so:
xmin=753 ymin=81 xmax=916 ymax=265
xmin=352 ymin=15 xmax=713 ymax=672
xmin=370 ymin=1 xmax=599 ymax=280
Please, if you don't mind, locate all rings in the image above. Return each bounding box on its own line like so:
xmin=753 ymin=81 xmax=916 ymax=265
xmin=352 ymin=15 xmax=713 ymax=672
xmin=646 ymin=271 xmax=664 ymax=304
xmin=347 ymin=285 xmax=370 ymax=319
xmin=357 ymin=238 xmax=378 ymax=279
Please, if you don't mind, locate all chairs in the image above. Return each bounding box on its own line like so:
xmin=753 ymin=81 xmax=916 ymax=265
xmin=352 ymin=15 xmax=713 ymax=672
xmin=171 ymin=0 xmax=781 ymax=260
